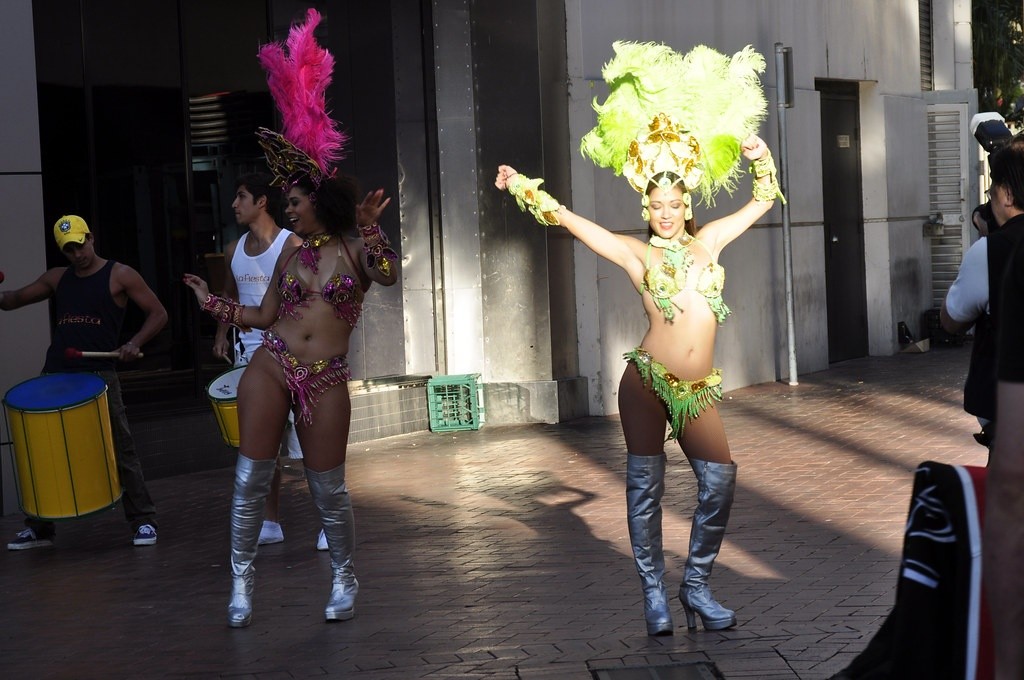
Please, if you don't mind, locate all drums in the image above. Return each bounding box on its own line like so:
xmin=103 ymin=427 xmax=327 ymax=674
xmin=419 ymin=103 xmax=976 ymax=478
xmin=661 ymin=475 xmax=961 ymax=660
xmin=206 ymin=367 xmax=248 ymax=450
xmin=4 ymin=370 xmax=125 ymax=522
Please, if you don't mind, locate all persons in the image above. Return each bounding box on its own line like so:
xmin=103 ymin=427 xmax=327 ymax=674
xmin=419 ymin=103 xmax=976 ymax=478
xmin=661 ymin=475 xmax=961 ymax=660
xmin=0 ymin=215 xmax=168 ymax=549
xmin=182 ymin=167 xmax=398 ymax=628
xmin=496 ymin=133 xmax=788 ymax=637
xmin=940 ymin=133 xmax=1024 ymax=468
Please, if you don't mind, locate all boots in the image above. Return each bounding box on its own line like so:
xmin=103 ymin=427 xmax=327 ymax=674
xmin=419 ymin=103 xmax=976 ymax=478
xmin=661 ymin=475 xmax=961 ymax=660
xmin=625 ymin=452 xmax=674 ymax=637
xmin=678 ymin=458 xmax=738 ymax=630
xmin=984 ymin=188 xmax=995 ymax=201
xmin=302 ymin=461 xmax=360 ymax=622
xmin=226 ymin=452 xmax=279 ymax=628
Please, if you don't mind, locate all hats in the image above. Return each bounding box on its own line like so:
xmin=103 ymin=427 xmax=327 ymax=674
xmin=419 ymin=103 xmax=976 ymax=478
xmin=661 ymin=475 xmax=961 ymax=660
xmin=53 ymin=215 xmax=91 ymax=251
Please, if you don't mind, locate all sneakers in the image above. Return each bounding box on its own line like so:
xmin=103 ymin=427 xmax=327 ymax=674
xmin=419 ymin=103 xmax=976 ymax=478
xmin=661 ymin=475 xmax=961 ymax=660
xmin=256 ymin=520 xmax=284 ymax=546
xmin=133 ymin=524 xmax=157 ymax=546
xmin=316 ymin=528 xmax=329 ymax=550
xmin=7 ymin=526 xmax=55 ymax=550
